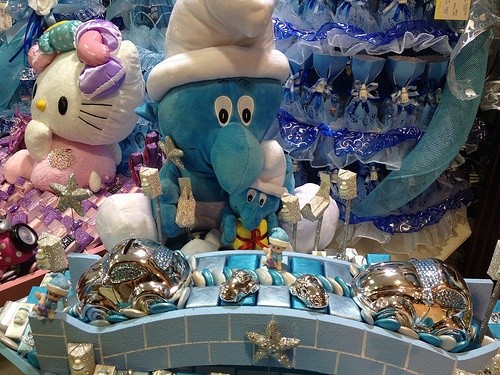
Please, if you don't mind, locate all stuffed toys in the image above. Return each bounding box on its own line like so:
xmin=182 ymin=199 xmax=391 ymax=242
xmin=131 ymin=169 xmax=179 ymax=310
xmin=179 ymin=140 xmax=295 ymax=258
xmin=3 ymin=20 xmax=145 ymax=194
xmin=134 ymin=0 xmax=339 ymax=254
